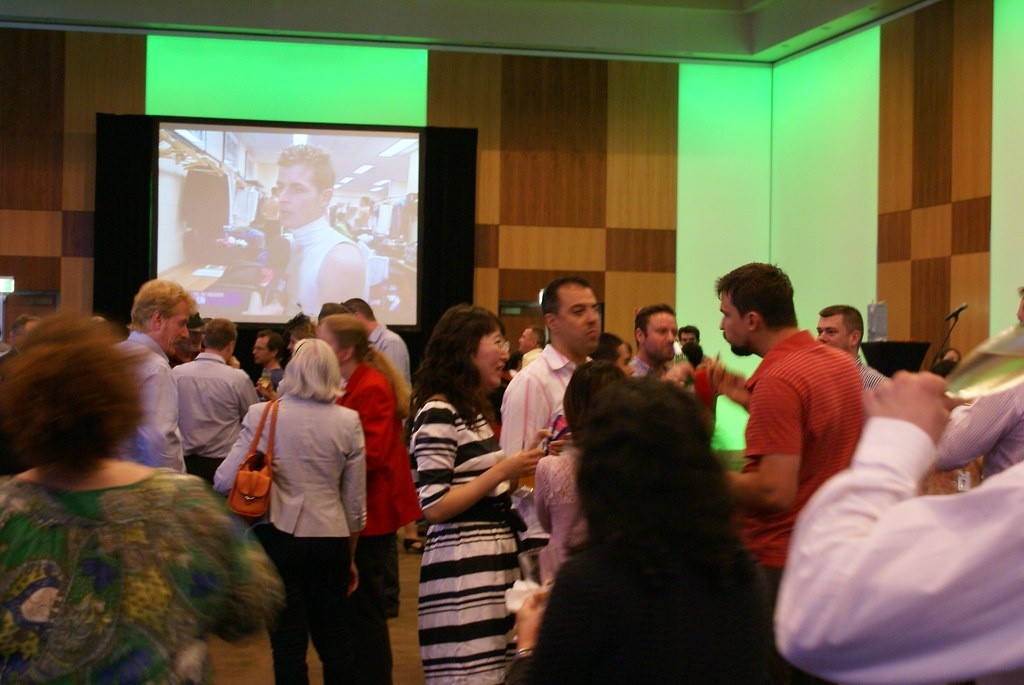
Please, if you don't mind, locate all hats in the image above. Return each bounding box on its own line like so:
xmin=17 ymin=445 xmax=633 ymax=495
xmin=282 ymin=313 xmax=310 ymax=336
xmin=186 ymin=313 xmax=205 ymax=332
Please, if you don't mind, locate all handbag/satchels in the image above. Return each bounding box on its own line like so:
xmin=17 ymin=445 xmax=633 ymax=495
xmin=226 ymin=400 xmax=280 ymax=523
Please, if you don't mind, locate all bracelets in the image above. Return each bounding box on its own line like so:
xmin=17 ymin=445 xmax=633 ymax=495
xmin=515 ymin=647 xmax=538 ymax=658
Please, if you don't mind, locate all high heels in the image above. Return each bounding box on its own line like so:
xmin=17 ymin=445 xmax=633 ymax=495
xmin=403 ymin=538 xmax=425 ymax=554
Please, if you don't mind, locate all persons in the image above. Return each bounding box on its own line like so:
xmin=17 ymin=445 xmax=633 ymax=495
xmin=105 ymin=276 xmax=195 ymax=475
xmin=259 ymin=144 xmax=416 ymax=321
xmin=160 ymin=298 xmax=410 ymax=449
xmin=929 ymin=284 xmax=1024 ymax=491
xmin=0 ymin=314 xmax=43 ymax=365
xmin=818 ymin=304 xmax=890 ymax=393
xmin=496 ymin=275 xmax=602 ymax=586
xmin=173 ymin=319 xmax=258 ymax=485
xmin=701 ymin=263 xmax=866 ymax=614
xmin=214 ymin=338 xmax=367 ymax=685
xmin=0 ymin=313 xmax=286 ymax=685
xmin=771 ymin=369 xmax=1024 ymax=685
xmin=314 ymin=314 xmax=424 ymax=685
xmin=492 ymin=297 xmax=751 ymax=457
xmin=534 ymin=358 xmax=628 ymax=587
xmin=497 ymin=376 xmax=791 ymax=684
xmin=403 ymin=303 xmax=566 ymax=685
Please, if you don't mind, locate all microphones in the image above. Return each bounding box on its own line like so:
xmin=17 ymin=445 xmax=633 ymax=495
xmin=944 ymin=303 xmax=969 ymax=321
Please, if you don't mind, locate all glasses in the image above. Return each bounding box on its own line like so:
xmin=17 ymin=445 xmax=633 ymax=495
xmin=478 ymin=341 xmax=510 ymax=353
